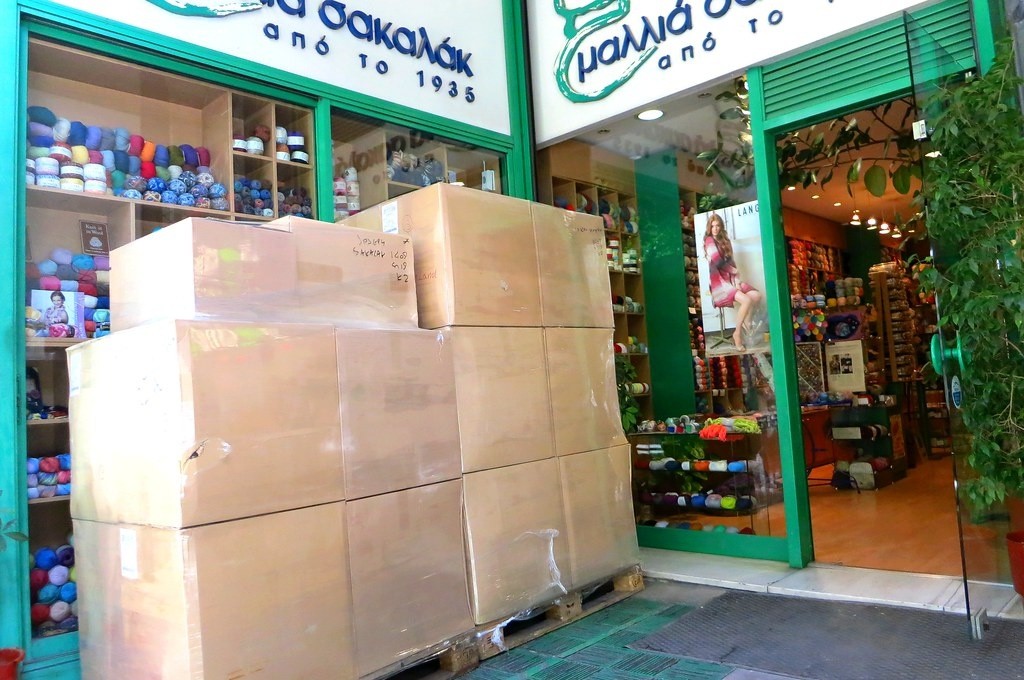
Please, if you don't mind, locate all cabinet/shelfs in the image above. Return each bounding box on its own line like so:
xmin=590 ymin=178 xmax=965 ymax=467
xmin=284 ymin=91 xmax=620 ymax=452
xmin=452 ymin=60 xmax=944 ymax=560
xmin=329 ymin=112 xmax=506 ymax=223
xmin=26 ymin=35 xmax=318 ymax=640
xmin=533 ymin=176 xmax=951 ymax=536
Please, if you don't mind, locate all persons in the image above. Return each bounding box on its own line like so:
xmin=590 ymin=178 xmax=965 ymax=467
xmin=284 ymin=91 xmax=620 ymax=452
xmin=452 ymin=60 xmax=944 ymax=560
xmin=703 ymin=214 xmax=762 ymax=352
xmin=36 ymin=291 xmax=68 ymax=336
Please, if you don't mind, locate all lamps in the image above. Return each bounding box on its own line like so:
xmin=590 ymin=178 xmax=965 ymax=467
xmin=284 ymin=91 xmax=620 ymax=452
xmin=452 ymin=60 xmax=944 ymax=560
xmin=891 ymin=213 xmax=901 ymax=238
xmin=849 ymin=183 xmax=861 ymax=225
xmin=865 ymin=192 xmax=877 ymax=230
xmin=878 ymin=197 xmax=890 ymax=234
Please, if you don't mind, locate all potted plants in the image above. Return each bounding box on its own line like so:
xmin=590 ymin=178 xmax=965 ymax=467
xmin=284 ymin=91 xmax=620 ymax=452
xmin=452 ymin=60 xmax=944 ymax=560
xmin=893 ymin=21 xmax=1024 ymax=600
xmin=0 ymin=490 xmax=28 ymax=680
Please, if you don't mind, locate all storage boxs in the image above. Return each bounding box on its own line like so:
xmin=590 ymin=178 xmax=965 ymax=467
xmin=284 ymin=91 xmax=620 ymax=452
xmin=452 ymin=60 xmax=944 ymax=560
xmin=64 ymin=178 xmax=640 ymax=680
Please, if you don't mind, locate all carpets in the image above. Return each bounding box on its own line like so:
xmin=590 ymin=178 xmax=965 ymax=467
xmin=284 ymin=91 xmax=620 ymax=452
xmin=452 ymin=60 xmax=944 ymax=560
xmin=622 ymin=590 xmax=1023 ymax=680
